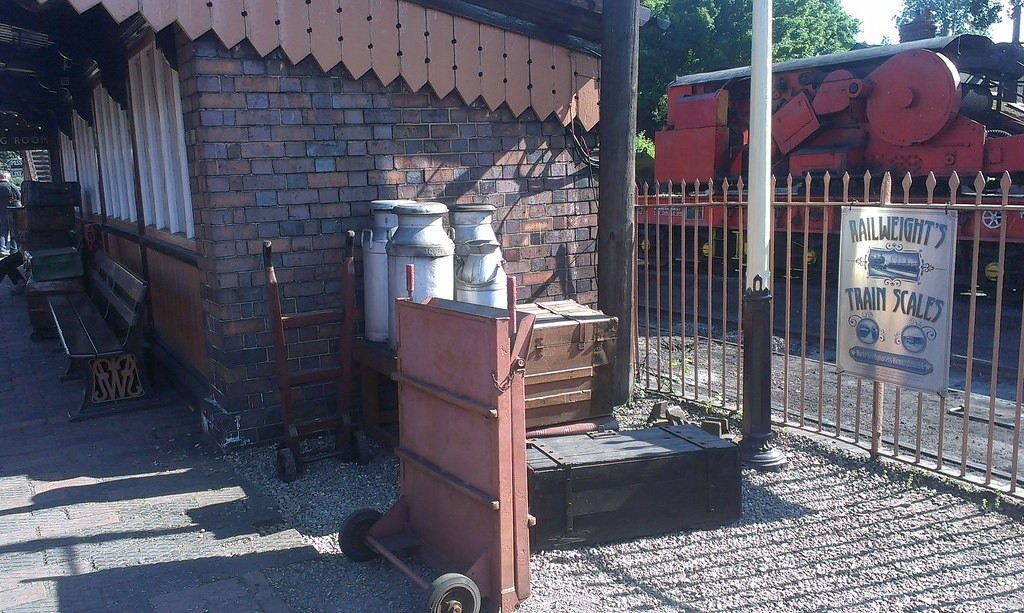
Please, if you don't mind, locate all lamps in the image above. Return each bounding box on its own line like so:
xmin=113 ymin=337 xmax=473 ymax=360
xmin=33 ymin=78 xmax=71 ymax=99
xmin=57 ymin=48 xmax=98 ymax=69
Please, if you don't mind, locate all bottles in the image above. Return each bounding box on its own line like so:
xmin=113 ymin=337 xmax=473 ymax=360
xmin=361 ymin=200 xmax=508 ymax=343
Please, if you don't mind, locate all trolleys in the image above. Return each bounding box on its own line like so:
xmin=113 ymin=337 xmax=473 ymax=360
xmin=262 ymin=239 xmax=371 ymax=482
xmin=339 ymin=264 xmax=538 ymax=613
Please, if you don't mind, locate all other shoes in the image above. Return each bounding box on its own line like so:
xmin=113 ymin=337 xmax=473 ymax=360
xmin=11 ymin=279 xmax=27 ymax=294
xmin=0 ymin=251 xmax=5 ymax=258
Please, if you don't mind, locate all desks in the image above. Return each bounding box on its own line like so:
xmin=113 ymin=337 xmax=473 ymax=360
xmin=25 ymin=277 xmax=85 ymax=341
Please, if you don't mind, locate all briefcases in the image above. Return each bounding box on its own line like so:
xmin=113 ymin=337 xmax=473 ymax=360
xmin=21 ymin=181 xmax=81 ymax=207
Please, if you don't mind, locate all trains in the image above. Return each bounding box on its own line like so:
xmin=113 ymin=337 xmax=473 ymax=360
xmin=638 ymin=4 xmax=1024 ymax=301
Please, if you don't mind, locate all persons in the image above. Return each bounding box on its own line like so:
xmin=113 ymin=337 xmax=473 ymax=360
xmin=0 ymin=252 xmax=27 ymax=292
xmin=0 ymin=170 xmax=21 ymax=255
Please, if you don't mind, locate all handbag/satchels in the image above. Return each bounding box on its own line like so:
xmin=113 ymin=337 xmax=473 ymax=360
xmin=11 ymin=183 xmax=22 ymax=208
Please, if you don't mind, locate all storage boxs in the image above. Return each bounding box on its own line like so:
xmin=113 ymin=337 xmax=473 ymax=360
xmin=14 ymin=180 xmax=83 ymax=281
xmin=514 ymin=300 xmax=742 ymax=550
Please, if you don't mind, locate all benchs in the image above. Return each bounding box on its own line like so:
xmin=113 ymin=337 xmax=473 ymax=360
xmin=48 ymin=248 xmax=161 ymax=421
